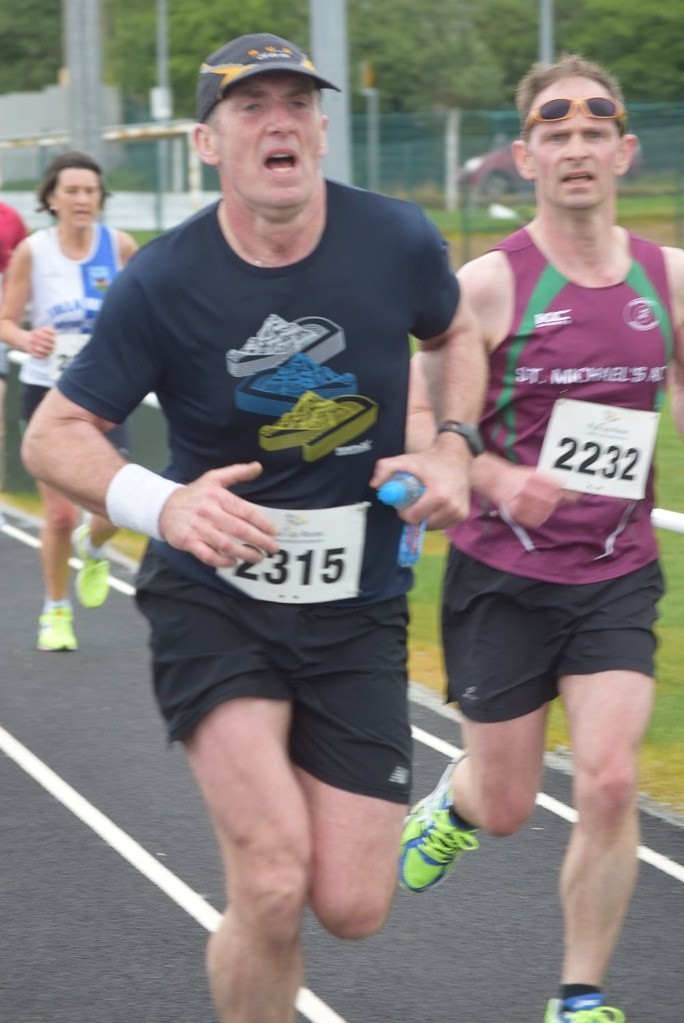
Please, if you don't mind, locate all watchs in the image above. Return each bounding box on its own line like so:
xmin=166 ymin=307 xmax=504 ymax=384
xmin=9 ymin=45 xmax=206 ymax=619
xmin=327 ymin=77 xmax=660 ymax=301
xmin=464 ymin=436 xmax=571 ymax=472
xmin=439 ymin=419 xmax=484 ymax=457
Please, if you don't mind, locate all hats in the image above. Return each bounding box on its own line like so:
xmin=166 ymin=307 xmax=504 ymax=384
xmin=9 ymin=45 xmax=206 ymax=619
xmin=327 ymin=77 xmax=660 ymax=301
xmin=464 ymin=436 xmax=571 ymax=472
xmin=195 ymin=32 xmax=343 ymax=123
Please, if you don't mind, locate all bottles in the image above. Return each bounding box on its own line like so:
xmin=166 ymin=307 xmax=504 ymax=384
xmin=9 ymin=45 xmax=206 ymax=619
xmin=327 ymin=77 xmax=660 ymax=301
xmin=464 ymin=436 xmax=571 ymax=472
xmin=375 ymin=471 xmax=424 ymax=511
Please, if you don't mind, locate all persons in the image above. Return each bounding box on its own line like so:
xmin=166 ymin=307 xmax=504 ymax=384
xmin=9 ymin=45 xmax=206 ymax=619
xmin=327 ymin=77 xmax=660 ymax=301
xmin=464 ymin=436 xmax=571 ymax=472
xmin=399 ymin=59 xmax=683 ymax=1022
xmin=0 ymin=151 xmax=144 ymax=652
xmin=18 ymin=31 xmax=487 ymax=1023
xmin=0 ymin=202 xmax=29 ymax=493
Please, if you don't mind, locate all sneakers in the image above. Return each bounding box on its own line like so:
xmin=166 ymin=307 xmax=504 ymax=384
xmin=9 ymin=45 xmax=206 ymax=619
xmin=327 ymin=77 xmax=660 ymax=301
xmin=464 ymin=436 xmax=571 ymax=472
xmin=398 ymin=752 xmax=481 ymax=892
xmin=35 ymin=607 xmax=79 ymax=652
xmin=545 ymin=993 xmax=626 ymax=1023
xmin=72 ymin=524 xmax=111 ymax=609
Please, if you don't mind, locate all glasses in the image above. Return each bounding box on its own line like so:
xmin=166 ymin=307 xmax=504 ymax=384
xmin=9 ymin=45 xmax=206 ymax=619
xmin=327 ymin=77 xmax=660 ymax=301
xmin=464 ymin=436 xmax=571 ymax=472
xmin=522 ymin=96 xmax=625 ymax=139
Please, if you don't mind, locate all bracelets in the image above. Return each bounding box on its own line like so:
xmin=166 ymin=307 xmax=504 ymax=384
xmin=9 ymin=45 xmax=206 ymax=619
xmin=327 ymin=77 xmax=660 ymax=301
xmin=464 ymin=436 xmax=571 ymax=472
xmin=106 ymin=464 xmax=184 ymax=544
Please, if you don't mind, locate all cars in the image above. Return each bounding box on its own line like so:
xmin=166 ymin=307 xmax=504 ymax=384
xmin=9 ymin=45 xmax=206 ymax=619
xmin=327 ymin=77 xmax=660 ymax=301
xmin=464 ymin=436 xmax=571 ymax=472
xmin=463 ymin=135 xmax=645 ymax=213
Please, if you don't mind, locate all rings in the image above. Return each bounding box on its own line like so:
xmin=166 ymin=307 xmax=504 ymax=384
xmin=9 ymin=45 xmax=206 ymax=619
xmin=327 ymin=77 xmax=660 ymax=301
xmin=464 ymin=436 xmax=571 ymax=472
xmin=35 ymin=346 xmax=43 ymax=352
